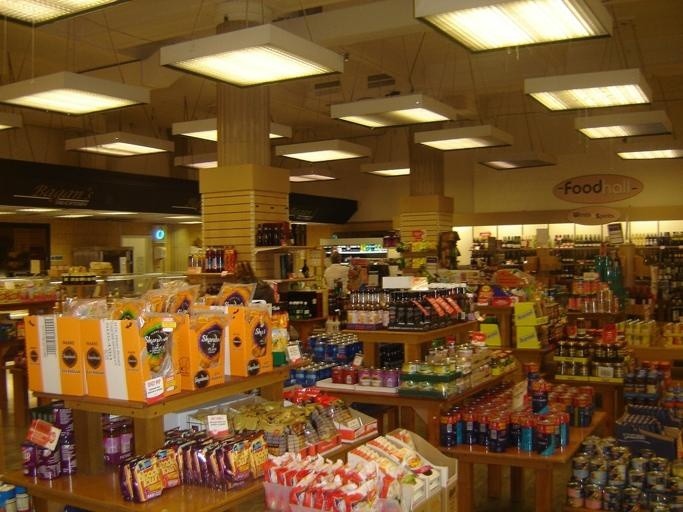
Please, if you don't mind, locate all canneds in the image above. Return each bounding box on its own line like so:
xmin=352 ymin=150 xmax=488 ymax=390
xmin=398 ymin=331 xmax=514 ymax=395
xmin=283 ymin=332 xmax=362 ymax=387
xmin=438 ymin=361 xmax=595 ymax=456
xmin=564 ymin=434 xmax=683 ymax=511
xmin=557 ymin=327 xmax=636 ymax=381
xmin=580 ymin=289 xmax=619 ymax=315
xmin=620 ymin=361 xmax=682 ymax=427
xmin=331 ymin=363 xmax=399 ymax=390
xmin=615 ymin=318 xmax=683 ymax=348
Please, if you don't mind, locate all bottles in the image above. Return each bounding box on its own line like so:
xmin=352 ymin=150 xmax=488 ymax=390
xmin=205 ymin=245 xmax=237 ymax=272
xmin=659 ymin=231 xmax=683 ymax=246
xmin=346 ymin=289 xmax=390 ymax=327
xmin=502 ymin=236 xmax=521 ymax=248
xmin=390 ymin=287 xmax=469 ymax=327
xmin=289 ymin=301 xmax=310 ymax=320
xmin=302 ymin=259 xmax=309 ymax=278
xmin=379 ymin=351 xmax=404 ymax=369
xmin=256 ymin=222 xmax=283 ymax=246
xmin=575 ymin=234 xmax=600 ymax=247
xmin=554 ymin=235 xmax=574 ymax=249
xmin=632 ymin=234 xmax=657 ymax=245
xmin=561 ymin=250 xmax=596 ymax=264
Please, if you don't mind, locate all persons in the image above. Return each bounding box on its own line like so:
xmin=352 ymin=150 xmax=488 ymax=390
xmin=324 ymin=251 xmax=353 ymax=289
